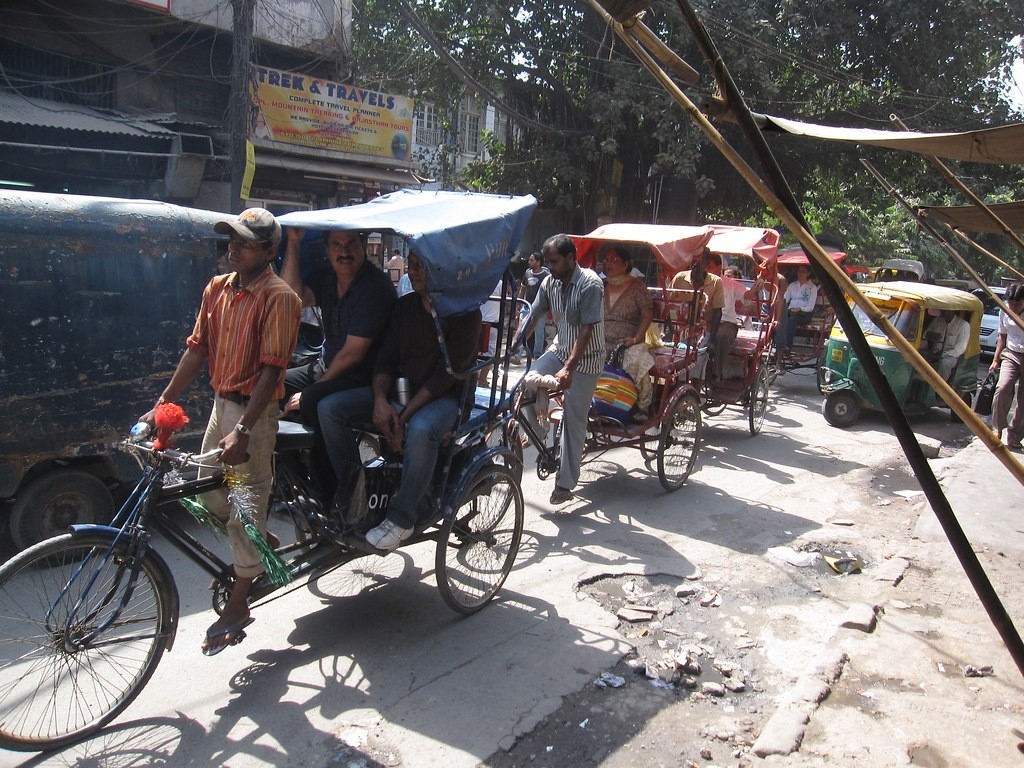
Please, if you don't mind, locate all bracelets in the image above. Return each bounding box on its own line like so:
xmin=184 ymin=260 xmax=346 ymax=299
xmin=633 ymin=338 xmax=635 ymax=344
xmin=993 ymin=359 xmax=1000 ymax=365
xmin=159 ymin=396 xmax=176 ymax=405
xmin=236 ymin=423 xmax=251 ymax=435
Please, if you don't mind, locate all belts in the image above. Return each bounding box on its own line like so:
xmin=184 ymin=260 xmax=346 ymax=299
xmin=219 ymin=392 xmax=250 ymax=402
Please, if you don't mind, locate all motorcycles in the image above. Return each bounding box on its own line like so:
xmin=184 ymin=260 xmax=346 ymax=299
xmin=0 ymin=187 xmax=240 ymax=570
xmin=818 ymin=281 xmax=985 ymax=428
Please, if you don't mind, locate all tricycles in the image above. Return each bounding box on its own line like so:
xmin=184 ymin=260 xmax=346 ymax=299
xmin=639 ymin=224 xmax=924 ymax=460
xmin=0 ymin=187 xmax=540 ymax=755
xmin=457 ymin=224 xmax=714 ymax=535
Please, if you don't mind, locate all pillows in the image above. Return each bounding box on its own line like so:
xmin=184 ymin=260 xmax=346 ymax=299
xmin=590 ymin=364 xmax=639 ymax=423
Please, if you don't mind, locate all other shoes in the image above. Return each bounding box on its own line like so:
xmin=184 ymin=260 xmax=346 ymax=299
xmin=510 ymin=356 xmax=520 ymax=364
xmin=775 ymin=365 xmax=786 ymax=375
xmin=711 ymin=375 xmax=725 ymax=388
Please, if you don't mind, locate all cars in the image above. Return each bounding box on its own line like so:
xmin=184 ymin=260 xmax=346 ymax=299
xmin=970 ymin=286 xmax=1007 ymax=354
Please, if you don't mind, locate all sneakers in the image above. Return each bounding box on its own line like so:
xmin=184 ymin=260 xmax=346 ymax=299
xmin=366 ymin=518 xmax=414 ymax=549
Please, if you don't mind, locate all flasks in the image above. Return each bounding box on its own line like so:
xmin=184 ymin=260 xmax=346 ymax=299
xmin=395 ymin=377 xmax=410 ymax=407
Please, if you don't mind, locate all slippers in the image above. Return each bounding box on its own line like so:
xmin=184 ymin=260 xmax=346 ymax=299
xmin=478 ymin=382 xmax=490 ymax=387
xmin=519 ymin=432 xmax=547 ymax=449
xmin=550 ymin=489 xmax=572 ymax=504
xmin=1007 ymin=436 xmax=1024 ymax=449
xmin=202 ymin=617 xmax=255 ymax=656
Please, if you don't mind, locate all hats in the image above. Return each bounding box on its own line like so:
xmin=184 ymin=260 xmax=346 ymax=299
xmin=214 ymin=207 xmax=282 ymax=249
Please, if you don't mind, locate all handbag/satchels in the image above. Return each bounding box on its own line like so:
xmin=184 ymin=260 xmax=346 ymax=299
xmin=974 ymin=368 xmax=998 ymax=415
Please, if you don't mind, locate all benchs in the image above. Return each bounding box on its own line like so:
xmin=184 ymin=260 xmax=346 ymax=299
xmin=279 ymin=357 xmax=512 ymax=513
xmin=726 ymin=277 xmax=778 ymax=388
xmin=796 ymin=300 xmax=835 ymax=353
xmin=639 ymin=288 xmax=709 ymax=416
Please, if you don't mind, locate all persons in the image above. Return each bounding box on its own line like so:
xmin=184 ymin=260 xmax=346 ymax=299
xmin=912 ymin=310 xmax=970 ymax=408
xmin=478 ymin=252 xmax=551 ymax=388
xmin=988 ymin=283 xmax=1024 ymax=451
xmin=850 ymin=269 xmax=936 ymax=285
xmin=666 ymin=247 xmax=817 ymax=406
xmin=279 ymin=227 xmax=483 ymax=549
xmin=595 ymin=247 xmax=657 ymax=421
xmin=139 ymin=207 xmax=303 ymax=656
xmin=512 ymin=234 xmax=607 ymax=504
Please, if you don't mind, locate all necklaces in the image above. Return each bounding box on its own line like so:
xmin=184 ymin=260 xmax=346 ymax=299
xmin=236 ymin=282 xmax=247 ymax=288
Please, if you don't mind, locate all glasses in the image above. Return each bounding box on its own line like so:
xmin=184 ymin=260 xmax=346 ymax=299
xmin=229 ymin=235 xmax=271 ymax=250
xmin=603 ymin=257 xmax=626 ymax=265
